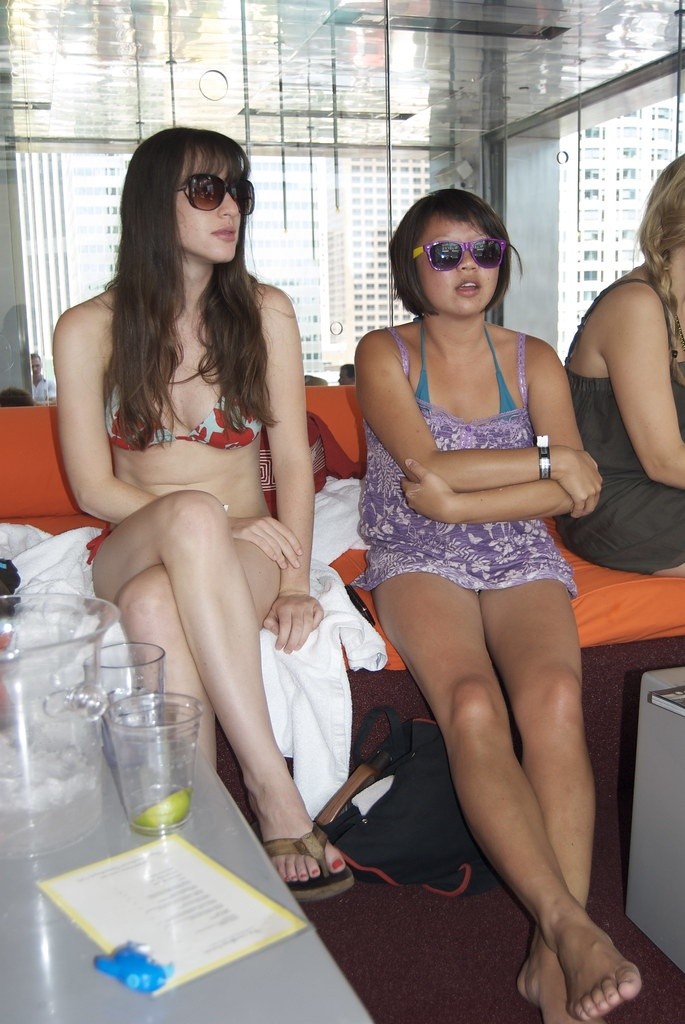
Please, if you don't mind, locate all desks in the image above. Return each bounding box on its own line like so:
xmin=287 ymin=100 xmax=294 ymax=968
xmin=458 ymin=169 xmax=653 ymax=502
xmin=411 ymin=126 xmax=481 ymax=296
xmin=1 ymin=743 xmax=372 ymax=1024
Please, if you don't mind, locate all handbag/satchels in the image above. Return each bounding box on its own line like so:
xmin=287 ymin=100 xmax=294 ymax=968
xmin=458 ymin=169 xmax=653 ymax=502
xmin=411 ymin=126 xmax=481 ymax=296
xmin=313 ymin=705 xmax=523 ymax=898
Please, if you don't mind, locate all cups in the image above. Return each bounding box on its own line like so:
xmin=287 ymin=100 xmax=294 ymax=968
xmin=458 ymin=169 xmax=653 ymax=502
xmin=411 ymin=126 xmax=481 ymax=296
xmin=85 ymin=643 xmax=164 ymax=766
xmin=0 ymin=594 xmax=119 ymax=858
xmin=104 ymin=693 xmax=203 ymax=833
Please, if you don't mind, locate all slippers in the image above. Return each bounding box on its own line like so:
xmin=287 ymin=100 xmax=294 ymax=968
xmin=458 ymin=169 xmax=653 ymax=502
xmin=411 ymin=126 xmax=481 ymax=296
xmin=250 ymin=819 xmax=356 ymax=903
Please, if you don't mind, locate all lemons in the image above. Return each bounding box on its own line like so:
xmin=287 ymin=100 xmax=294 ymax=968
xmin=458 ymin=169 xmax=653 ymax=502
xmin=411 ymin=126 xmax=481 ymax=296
xmin=133 ymin=787 xmax=193 ymax=828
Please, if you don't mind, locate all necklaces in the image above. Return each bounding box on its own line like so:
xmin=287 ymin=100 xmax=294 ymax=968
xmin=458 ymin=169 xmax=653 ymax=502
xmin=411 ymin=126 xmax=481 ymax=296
xmin=674 ymin=312 xmax=685 ymax=353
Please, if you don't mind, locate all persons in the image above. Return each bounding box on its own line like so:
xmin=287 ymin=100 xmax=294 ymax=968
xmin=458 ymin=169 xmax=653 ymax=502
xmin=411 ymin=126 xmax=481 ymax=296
xmin=338 ymin=363 xmax=356 ymax=388
xmin=554 ymin=154 xmax=685 ymax=578
xmin=51 ymin=126 xmax=354 ymax=901
xmin=351 ymin=187 xmax=642 ymax=1024
xmin=30 ymin=352 xmax=57 ymax=403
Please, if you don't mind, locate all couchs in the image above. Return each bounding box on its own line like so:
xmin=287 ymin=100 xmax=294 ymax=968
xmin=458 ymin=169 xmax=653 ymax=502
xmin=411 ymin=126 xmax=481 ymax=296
xmin=1 ymin=383 xmax=685 ymax=672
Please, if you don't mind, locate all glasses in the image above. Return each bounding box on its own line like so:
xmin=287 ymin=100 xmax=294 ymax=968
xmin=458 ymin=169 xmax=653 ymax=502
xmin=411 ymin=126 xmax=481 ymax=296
xmin=413 ymin=238 xmax=506 ymax=271
xmin=173 ymin=173 xmax=255 ymax=215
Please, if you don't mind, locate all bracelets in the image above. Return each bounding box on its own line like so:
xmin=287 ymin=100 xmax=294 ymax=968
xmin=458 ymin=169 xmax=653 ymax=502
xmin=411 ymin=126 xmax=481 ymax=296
xmin=536 ymin=435 xmax=551 ymax=481
